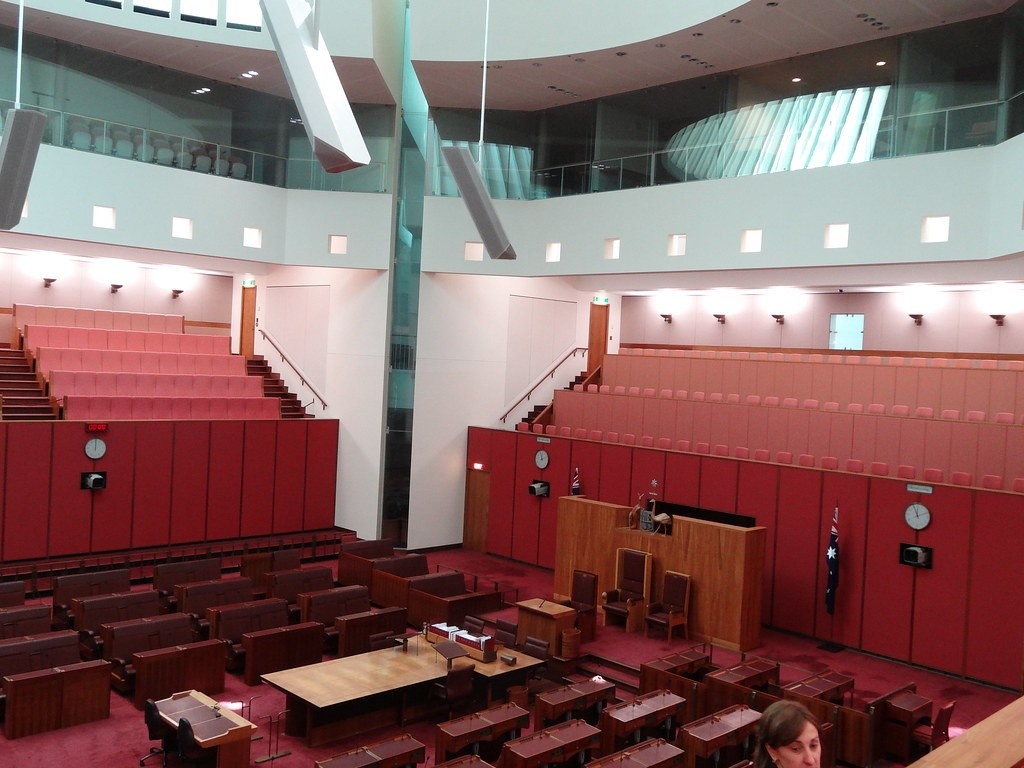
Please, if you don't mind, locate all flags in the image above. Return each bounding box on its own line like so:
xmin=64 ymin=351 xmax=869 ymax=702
xmin=821 ymin=509 xmax=841 ymax=616
xmin=571 ymin=470 xmax=582 ymax=494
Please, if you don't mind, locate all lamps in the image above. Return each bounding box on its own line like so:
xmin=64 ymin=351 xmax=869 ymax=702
xmin=714 ymin=314 xmax=725 ymax=324
xmin=772 ymin=314 xmax=784 ymax=325
xmin=43 ymin=279 xmax=56 ymax=288
xmin=661 ymin=314 xmax=672 ymax=323
xmin=989 ymin=315 xmax=1006 ymax=326
xmin=111 ymin=283 xmax=123 ymax=294
xmin=172 ymin=289 xmax=184 ymax=299
xmin=909 ymin=314 xmax=923 ymax=326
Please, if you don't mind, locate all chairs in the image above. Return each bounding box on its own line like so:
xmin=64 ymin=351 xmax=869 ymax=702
xmin=432 ymin=663 xmax=475 ymax=720
xmin=12 ymin=304 xmax=281 ymax=421
xmin=368 ymin=629 xmax=394 ymax=651
xmin=139 ymin=698 xmax=178 ymax=768
xmin=493 ymin=617 xmax=518 ymax=650
xmin=21 ymin=105 xmax=248 ymax=182
xmin=523 ymin=634 xmax=551 ymax=681
xmin=601 ymin=547 xmax=653 ymax=632
xmin=560 ymin=569 xmax=598 ymax=644
xmin=913 ymin=700 xmax=957 ymax=751
xmin=518 ymin=348 xmax=1024 ymax=495
xmin=176 ymin=718 xmax=212 ymax=768
xmin=645 ymin=570 xmax=691 ymax=650
xmin=463 ymin=615 xmax=485 ymax=636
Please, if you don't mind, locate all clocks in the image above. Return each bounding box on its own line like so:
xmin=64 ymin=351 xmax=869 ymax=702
xmin=84 ymin=438 xmax=106 ymax=460
xmin=534 ymin=450 xmax=550 ymax=469
xmin=905 ymin=502 xmax=930 ymax=531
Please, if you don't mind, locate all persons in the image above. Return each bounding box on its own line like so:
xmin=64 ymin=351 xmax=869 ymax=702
xmin=749 ymin=699 xmax=823 ymax=767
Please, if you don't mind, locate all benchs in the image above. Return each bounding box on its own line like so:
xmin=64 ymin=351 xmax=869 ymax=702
xmin=0 ymin=538 xmax=502 ymax=742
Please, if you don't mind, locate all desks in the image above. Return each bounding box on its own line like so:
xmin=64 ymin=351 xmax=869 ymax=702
xmin=155 ymin=597 xmax=960 ymax=768
